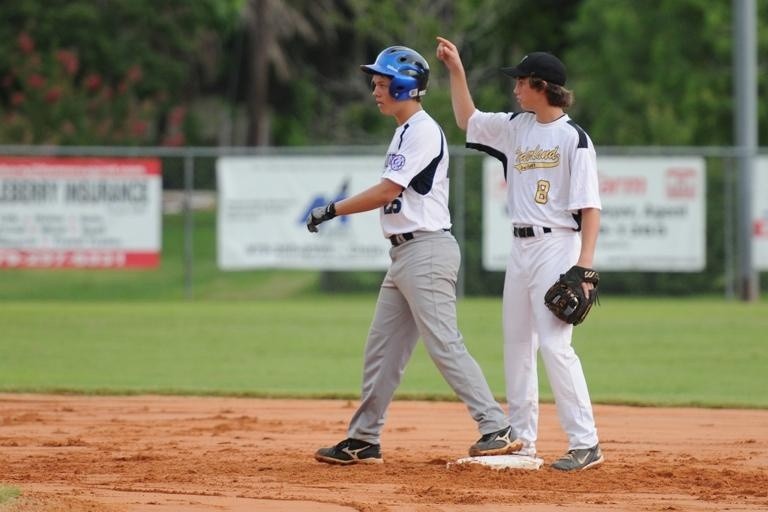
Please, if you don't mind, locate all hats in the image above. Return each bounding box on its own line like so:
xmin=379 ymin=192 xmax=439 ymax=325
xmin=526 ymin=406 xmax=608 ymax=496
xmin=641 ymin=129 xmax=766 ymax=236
xmin=499 ymin=50 xmax=568 ymax=86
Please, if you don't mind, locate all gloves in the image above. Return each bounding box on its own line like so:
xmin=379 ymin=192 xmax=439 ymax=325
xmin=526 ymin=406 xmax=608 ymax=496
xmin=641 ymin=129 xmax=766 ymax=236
xmin=304 ymin=200 xmax=337 ymax=233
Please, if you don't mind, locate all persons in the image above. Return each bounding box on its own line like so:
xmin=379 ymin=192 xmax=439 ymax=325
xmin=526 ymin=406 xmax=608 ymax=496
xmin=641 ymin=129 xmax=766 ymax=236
xmin=304 ymin=45 xmax=526 ymax=469
xmin=433 ymin=33 xmax=607 ymax=473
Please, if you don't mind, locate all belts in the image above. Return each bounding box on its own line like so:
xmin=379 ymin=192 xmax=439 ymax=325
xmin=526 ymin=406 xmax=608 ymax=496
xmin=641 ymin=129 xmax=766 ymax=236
xmin=389 ymin=227 xmax=451 ymax=247
xmin=515 ymin=224 xmax=553 ymax=238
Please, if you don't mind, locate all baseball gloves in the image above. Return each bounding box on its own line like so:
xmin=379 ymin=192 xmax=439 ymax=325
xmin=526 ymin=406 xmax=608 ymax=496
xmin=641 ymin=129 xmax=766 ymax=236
xmin=544 ymin=265 xmax=599 ymax=325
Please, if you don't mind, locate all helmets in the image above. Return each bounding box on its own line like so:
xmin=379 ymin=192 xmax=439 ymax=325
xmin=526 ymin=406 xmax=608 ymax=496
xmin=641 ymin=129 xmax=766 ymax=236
xmin=359 ymin=45 xmax=431 ymax=102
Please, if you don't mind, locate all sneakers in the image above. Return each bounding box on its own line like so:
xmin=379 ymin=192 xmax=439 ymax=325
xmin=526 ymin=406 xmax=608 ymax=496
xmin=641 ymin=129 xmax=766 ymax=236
xmin=314 ymin=436 xmax=385 ymax=466
xmin=549 ymin=440 xmax=605 ymax=475
xmin=467 ymin=425 xmax=525 ymax=457
